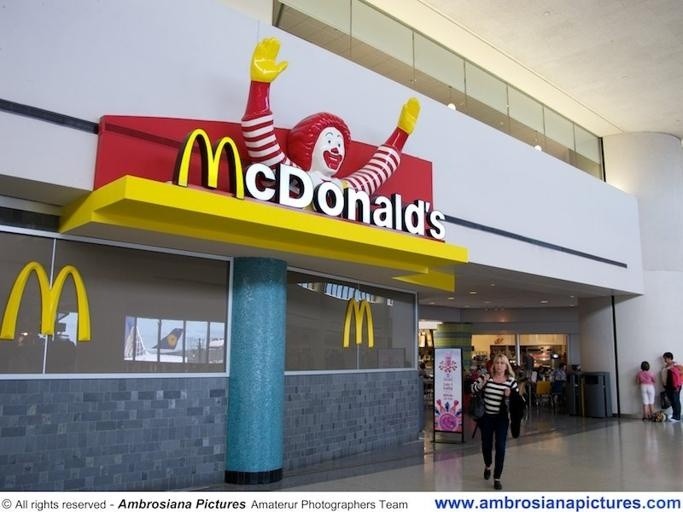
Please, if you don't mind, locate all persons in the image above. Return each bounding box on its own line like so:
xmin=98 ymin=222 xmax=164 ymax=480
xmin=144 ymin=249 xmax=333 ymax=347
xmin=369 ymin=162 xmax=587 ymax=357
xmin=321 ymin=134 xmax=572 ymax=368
xmin=239 ymin=35 xmax=420 ymax=218
xmin=660 ymin=352 xmax=682 ymax=422
xmin=418 ymin=361 xmax=430 ymax=394
xmin=476 ymin=348 xmax=566 ymax=380
xmin=469 ymin=353 xmax=518 ymax=489
xmin=636 ymin=361 xmax=656 ymax=420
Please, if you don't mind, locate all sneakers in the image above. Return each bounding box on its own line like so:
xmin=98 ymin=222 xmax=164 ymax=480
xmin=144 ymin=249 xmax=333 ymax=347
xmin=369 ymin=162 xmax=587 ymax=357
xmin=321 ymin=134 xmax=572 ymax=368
xmin=642 ymin=416 xmax=654 ymax=421
xmin=483 ymin=463 xmax=492 ymax=480
xmin=494 ymin=477 xmax=502 ymax=490
xmin=671 ymin=415 xmax=680 ymax=420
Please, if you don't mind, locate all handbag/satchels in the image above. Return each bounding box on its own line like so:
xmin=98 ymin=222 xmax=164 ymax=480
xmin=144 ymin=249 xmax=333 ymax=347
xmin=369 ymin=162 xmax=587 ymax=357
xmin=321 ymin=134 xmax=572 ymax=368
xmin=467 ymin=379 xmax=488 ymax=420
xmin=660 ymin=389 xmax=673 ymax=410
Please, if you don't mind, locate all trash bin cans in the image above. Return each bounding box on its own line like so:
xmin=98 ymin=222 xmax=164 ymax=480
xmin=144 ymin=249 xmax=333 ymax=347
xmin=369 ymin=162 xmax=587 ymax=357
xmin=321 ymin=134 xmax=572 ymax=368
xmin=582 ymin=371 xmax=613 ymax=418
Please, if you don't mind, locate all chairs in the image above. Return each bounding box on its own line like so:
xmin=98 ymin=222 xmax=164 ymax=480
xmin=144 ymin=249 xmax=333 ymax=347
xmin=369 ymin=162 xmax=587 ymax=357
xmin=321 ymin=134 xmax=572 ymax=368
xmin=534 ymin=380 xmax=567 ymax=416
